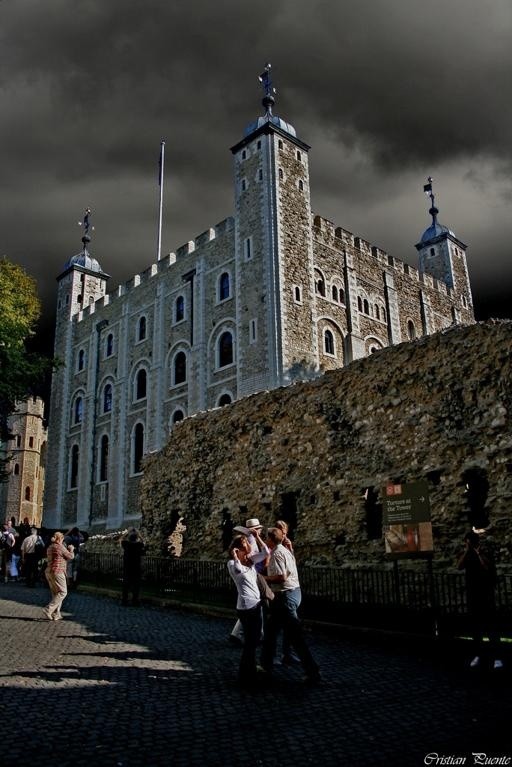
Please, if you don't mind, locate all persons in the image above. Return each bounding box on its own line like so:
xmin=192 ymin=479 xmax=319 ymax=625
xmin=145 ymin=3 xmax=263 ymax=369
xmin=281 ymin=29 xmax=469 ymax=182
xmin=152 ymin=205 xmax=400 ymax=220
xmin=0 ymin=520 xmax=85 ymax=621
xmin=455 ymin=531 xmax=504 ymax=669
xmin=226 ymin=518 xmax=322 ymax=688
xmin=118 ymin=528 xmax=145 ymax=606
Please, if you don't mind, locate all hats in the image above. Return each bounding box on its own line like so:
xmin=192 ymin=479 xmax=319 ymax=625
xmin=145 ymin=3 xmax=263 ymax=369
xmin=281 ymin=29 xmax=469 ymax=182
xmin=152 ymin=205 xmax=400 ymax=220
xmin=244 ymin=518 xmax=263 ymax=531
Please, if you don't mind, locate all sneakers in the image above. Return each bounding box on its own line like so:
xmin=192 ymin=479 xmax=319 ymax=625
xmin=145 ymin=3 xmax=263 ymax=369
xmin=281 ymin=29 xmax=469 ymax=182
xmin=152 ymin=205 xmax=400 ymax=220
xmin=229 ymin=634 xmax=242 ymax=646
xmin=41 ymin=608 xmax=64 ymax=622
xmin=247 ymin=653 xmax=323 ymax=688
